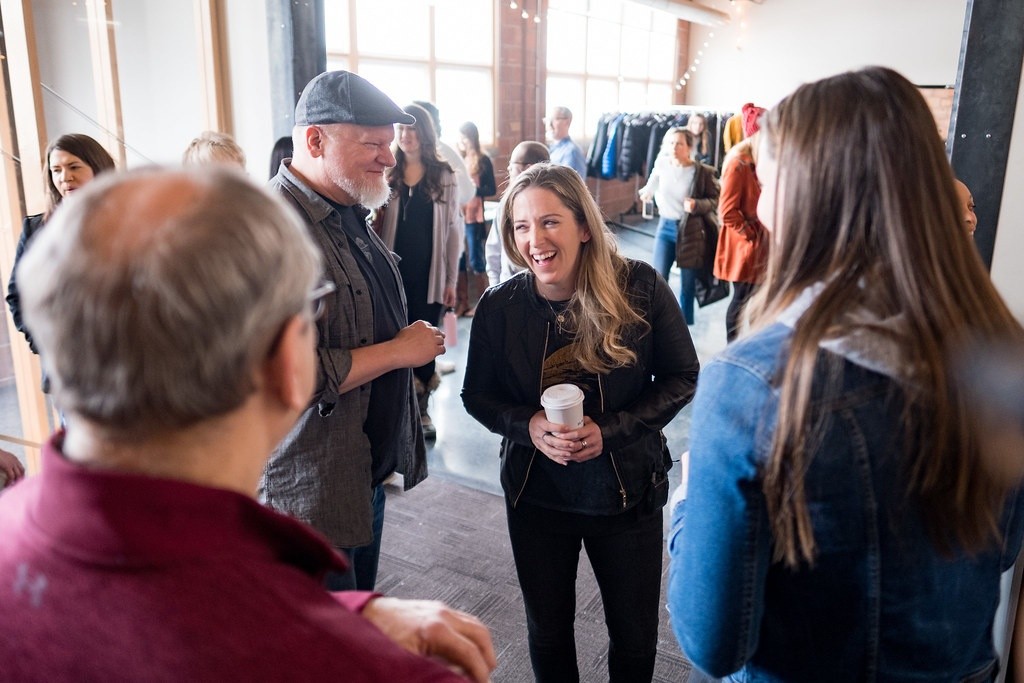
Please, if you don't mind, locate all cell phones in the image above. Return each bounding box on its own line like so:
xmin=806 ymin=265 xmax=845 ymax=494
xmin=643 ymin=200 xmax=654 ymax=220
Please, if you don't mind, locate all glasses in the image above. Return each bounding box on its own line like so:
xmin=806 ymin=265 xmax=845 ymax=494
xmin=543 ymin=117 xmax=567 ymax=124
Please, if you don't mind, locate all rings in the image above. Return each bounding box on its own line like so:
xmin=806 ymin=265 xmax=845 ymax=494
xmin=580 ymin=440 xmax=587 ymax=449
xmin=542 ymin=433 xmax=548 ymax=438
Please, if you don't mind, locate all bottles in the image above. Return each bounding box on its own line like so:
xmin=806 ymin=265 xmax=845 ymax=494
xmin=642 ymin=196 xmax=653 ymax=219
xmin=443 ymin=307 xmax=457 ymax=347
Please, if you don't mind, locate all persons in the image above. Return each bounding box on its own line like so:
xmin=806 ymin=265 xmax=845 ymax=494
xmin=485 ymin=141 xmax=550 ymax=287
xmin=710 ymin=102 xmax=769 ymax=344
xmin=269 ymin=137 xmax=293 ymax=178
xmin=668 ymin=65 xmax=1023 ymax=682
xmin=368 ymin=99 xmax=496 ymax=441
xmin=638 ymin=113 xmax=729 ymax=324
xmin=8 ymin=134 xmax=116 ymax=393
xmin=461 ymin=163 xmax=699 ymax=682
xmin=254 ymin=70 xmax=446 ymax=591
xmin=0 ymin=162 xmax=497 ymax=683
xmin=542 ymin=107 xmax=585 ymax=180
xmin=951 ymin=176 xmax=977 ymax=240
xmin=185 ymin=131 xmax=246 ymax=171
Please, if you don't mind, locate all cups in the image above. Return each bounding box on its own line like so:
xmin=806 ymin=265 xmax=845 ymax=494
xmin=540 ymin=384 xmax=584 ymax=437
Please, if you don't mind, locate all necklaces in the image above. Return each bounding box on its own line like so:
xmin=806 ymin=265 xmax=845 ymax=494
xmin=538 ymin=283 xmax=575 ymax=335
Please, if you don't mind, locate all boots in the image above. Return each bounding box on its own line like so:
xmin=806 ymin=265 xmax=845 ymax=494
xmin=415 ymin=374 xmax=441 ymax=438
xmin=455 ymin=272 xmax=468 ymax=316
xmin=464 ymin=272 xmax=487 ymax=317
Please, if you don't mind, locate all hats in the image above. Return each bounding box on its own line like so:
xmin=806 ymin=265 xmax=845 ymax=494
xmin=295 ymin=70 xmax=416 ymax=125
xmin=742 ymin=103 xmax=767 ymax=136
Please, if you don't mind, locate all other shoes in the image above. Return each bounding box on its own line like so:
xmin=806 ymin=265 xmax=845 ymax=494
xmin=436 ymin=360 xmax=455 ymax=373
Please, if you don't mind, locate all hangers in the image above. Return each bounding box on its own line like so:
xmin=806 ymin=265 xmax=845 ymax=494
xmin=599 ymin=109 xmax=713 ymax=130
